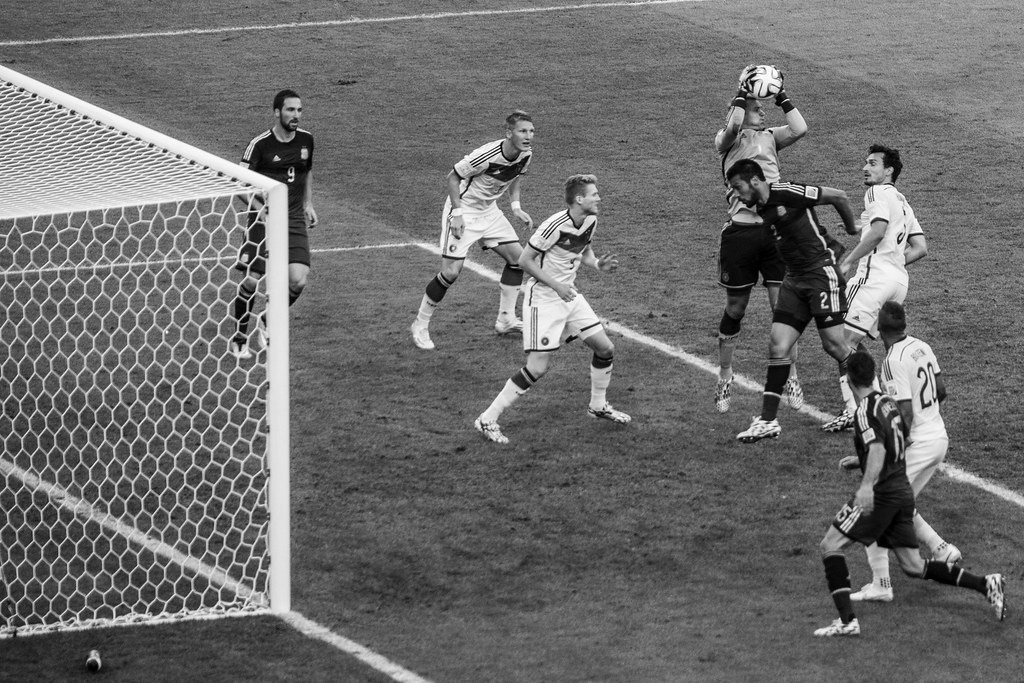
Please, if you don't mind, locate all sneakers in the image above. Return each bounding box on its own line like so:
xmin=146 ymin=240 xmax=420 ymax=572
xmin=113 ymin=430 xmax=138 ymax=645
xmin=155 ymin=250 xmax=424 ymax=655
xmin=495 ymin=317 xmax=525 ymax=337
xmin=258 ymin=318 xmax=269 ymax=346
xmin=587 ymin=402 xmax=632 ymax=422
xmin=814 ymin=615 xmax=862 ymax=635
xmin=930 ymin=544 xmax=962 ymax=565
xmin=474 ymin=413 xmax=509 ymax=445
xmin=736 ymin=415 xmax=782 ymax=443
xmin=786 ymin=374 xmax=804 ymax=410
xmin=231 ymin=340 xmax=251 ymax=359
xmin=411 ymin=323 xmax=435 ymax=351
xmin=820 ymin=409 xmax=858 ymax=433
xmin=714 ymin=375 xmax=734 ymax=414
xmin=850 ymin=583 xmax=894 ymax=602
xmin=984 ymin=573 xmax=1008 ymax=624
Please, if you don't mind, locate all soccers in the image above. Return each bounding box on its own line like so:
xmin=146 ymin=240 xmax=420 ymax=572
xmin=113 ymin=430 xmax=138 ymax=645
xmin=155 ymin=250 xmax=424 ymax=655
xmin=745 ymin=65 xmax=783 ymax=99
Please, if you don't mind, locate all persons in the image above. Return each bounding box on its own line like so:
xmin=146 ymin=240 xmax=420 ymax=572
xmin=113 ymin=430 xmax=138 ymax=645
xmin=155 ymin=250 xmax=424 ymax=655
xmin=473 ymin=174 xmax=631 ymax=443
xmin=848 ymin=302 xmax=964 ymax=600
xmin=714 ymin=64 xmax=807 ymax=413
xmin=811 ymin=352 xmax=1008 ymax=638
xmin=726 ymin=159 xmax=860 ymax=444
xmin=819 ymin=143 xmax=928 ymax=434
xmin=232 ymin=90 xmax=317 ymax=359
xmin=411 ymin=110 xmax=534 ymax=347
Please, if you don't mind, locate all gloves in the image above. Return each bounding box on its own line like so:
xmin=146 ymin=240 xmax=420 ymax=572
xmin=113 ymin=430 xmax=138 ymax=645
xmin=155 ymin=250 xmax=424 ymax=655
xmin=770 ymin=64 xmax=788 ymax=105
xmin=736 ymin=67 xmax=758 ymax=100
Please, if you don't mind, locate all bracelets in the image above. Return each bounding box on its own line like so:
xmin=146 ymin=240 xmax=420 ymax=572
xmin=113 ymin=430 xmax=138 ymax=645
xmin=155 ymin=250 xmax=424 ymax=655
xmin=731 ymin=99 xmax=748 ymax=109
xmin=511 ymin=201 xmax=521 ymax=209
xmin=781 ymin=100 xmax=795 ymax=112
xmin=594 ymin=259 xmax=599 ymax=270
xmin=452 ymin=209 xmax=462 ymax=216
